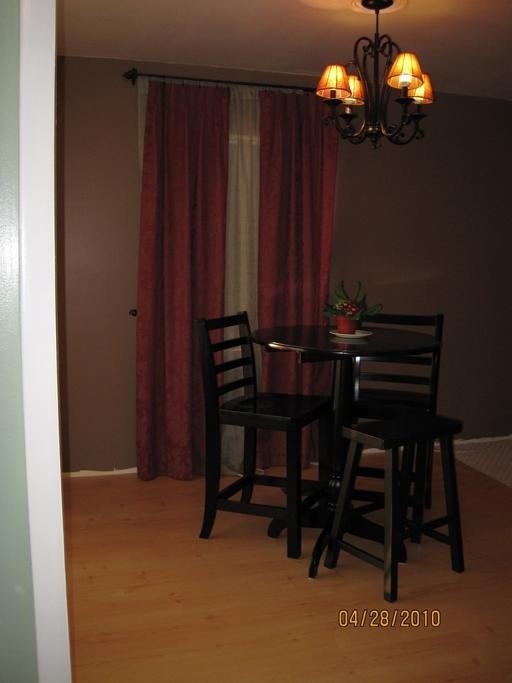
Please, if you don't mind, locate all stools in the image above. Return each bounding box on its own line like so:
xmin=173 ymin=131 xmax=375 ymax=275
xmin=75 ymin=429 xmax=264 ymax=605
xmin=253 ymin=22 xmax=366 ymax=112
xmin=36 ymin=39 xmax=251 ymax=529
xmin=325 ymin=415 xmax=464 ymax=601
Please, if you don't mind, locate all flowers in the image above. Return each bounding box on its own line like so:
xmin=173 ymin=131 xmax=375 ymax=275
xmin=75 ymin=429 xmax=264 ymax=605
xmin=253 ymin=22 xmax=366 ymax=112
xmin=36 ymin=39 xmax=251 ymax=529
xmin=323 ymin=280 xmax=383 ymax=323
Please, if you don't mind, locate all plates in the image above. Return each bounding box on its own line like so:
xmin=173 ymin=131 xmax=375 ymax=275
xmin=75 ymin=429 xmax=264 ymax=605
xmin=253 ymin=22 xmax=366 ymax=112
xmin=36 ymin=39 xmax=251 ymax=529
xmin=330 ymin=329 xmax=373 ymax=339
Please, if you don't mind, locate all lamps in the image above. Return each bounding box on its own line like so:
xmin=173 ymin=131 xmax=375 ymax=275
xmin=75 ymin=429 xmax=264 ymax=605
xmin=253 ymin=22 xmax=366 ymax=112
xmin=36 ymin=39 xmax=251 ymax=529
xmin=315 ymin=0 xmax=435 ymax=152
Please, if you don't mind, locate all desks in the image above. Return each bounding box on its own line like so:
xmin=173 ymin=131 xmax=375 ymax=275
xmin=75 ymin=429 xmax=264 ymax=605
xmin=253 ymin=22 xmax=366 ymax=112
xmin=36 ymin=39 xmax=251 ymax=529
xmin=243 ymin=326 xmax=437 ymax=546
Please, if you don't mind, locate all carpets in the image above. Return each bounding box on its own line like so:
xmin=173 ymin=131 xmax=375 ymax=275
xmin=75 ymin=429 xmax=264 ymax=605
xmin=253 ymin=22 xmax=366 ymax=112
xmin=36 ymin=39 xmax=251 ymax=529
xmin=449 ymin=439 xmax=511 ymax=489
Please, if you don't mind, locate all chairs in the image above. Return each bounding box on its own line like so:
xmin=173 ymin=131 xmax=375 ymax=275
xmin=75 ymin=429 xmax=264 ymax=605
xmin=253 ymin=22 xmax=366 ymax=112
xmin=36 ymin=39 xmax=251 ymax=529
xmin=336 ymin=310 xmax=444 ymax=544
xmin=194 ymin=310 xmax=333 ymax=558
